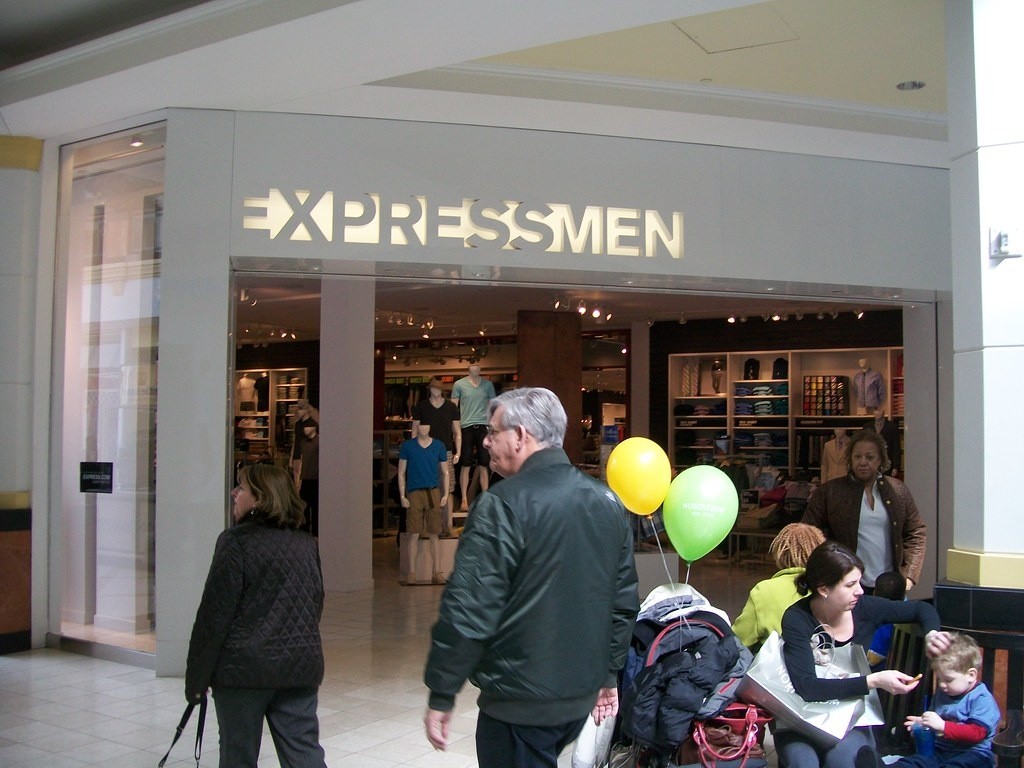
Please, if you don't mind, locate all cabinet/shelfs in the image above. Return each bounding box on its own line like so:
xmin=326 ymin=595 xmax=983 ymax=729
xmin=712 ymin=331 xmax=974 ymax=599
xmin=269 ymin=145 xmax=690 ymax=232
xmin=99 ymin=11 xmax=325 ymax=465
xmin=372 ymin=430 xmax=405 ymax=535
xmin=236 ymin=367 xmax=308 ymax=460
xmin=667 ymin=345 xmax=904 ymax=564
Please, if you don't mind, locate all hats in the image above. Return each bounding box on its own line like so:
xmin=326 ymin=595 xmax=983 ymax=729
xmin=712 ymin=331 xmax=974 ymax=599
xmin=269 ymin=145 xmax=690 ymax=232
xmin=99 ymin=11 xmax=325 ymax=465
xmin=426 ymin=377 xmax=447 ymax=390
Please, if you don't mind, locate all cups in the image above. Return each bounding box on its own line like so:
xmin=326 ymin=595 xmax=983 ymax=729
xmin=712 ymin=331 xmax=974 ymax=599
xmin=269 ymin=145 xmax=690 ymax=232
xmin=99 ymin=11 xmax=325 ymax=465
xmin=914 ymin=726 xmax=936 ymax=757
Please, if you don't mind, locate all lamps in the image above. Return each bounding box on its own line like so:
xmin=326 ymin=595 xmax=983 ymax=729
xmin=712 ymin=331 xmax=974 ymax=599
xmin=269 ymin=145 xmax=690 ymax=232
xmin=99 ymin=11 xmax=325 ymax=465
xmin=553 ymin=300 xmax=789 ymax=326
xmin=854 ymin=306 xmax=864 ymax=318
xmin=829 ymin=309 xmax=838 ymax=319
xmin=237 ymin=287 xmax=297 ymax=349
xmin=795 ymin=309 xmax=803 ymax=320
xmin=376 ymin=308 xmax=489 ymax=366
xmin=816 ymin=310 xmax=825 ymax=320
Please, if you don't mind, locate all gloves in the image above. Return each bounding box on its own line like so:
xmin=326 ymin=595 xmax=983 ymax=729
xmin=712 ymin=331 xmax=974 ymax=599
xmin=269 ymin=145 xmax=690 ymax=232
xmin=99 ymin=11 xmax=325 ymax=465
xmin=186 ymin=688 xmax=208 ymax=704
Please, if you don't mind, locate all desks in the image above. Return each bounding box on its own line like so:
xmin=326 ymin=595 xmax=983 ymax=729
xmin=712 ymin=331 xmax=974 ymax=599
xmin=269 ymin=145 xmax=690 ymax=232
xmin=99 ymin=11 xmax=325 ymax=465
xmin=728 ymin=528 xmax=780 ymax=572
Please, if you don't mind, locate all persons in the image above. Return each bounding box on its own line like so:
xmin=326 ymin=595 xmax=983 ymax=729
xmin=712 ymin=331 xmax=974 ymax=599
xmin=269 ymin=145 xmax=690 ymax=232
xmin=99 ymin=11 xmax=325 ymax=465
xmin=451 ymin=366 xmax=496 ymax=511
xmin=423 ymin=387 xmax=641 ymax=768
xmin=398 ymin=425 xmax=450 ymax=585
xmin=820 ymin=429 xmax=852 ymax=487
xmin=289 ymin=398 xmax=319 ymax=537
xmin=237 ymin=373 xmax=269 ymax=413
xmin=855 ymin=631 xmax=1001 ymax=768
xmin=731 ymin=523 xmax=827 ymax=646
xmin=864 ymin=572 xmax=909 ymax=673
xmin=800 ymin=427 xmax=927 ymax=596
xmin=852 ymin=359 xmax=886 ymax=415
xmin=185 ymin=457 xmax=326 ymax=768
xmin=862 ymin=410 xmax=901 ymax=477
xmin=412 ymin=374 xmax=462 ymax=528
xmin=772 ymin=540 xmax=956 ymax=768
xmin=711 ymin=361 xmax=723 ymax=394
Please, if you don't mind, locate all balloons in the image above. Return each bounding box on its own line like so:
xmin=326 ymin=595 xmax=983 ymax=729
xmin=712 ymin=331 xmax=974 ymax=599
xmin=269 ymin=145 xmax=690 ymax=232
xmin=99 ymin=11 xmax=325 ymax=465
xmin=663 ymin=465 xmax=739 ymax=565
xmin=606 ymin=437 xmax=672 ymax=519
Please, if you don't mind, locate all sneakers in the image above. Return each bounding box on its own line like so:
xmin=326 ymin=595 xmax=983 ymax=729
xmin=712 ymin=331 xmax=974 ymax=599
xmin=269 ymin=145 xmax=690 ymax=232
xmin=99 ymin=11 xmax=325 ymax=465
xmin=432 ymin=571 xmax=447 ymax=583
xmin=408 ymin=570 xmax=416 ymax=583
xmin=461 ymin=501 xmax=468 ymax=511
xmin=446 ymin=527 xmax=459 ymax=537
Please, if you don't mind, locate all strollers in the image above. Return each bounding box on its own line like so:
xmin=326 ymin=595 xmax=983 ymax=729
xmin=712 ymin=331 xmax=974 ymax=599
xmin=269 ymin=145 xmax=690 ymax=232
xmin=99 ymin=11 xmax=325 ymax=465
xmin=608 ymin=583 xmax=774 ymax=768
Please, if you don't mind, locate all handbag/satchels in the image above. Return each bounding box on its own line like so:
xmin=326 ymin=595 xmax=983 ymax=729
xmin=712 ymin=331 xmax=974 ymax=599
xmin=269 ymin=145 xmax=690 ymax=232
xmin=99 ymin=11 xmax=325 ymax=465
xmin=735 ymin=623 xmax=886 ymax=750
xmin=693 ymin=701 xmax=774 ymax=768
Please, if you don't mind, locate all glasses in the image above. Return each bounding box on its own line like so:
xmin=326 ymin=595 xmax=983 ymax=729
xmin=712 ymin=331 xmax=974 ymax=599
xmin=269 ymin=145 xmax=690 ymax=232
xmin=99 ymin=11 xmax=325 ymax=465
xmin=486 ymin=425 xmax=528 ymax=439
